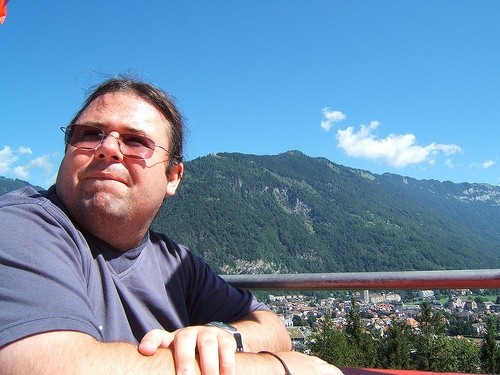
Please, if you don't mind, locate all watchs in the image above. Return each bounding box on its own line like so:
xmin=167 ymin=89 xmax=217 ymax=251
xmin=204 ymin=320 xmax=243 ymax=352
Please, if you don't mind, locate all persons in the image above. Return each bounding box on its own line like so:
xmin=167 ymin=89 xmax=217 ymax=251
xmin=0 ymin=70 xmax=346 ymax=375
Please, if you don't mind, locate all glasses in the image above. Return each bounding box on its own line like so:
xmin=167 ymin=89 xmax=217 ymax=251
xmin=60 ymin=124 xmax=173 ymax=159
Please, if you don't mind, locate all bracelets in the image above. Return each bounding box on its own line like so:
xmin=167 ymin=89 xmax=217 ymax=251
xmin=258 ymin=350 xmax=291 ymax=375
